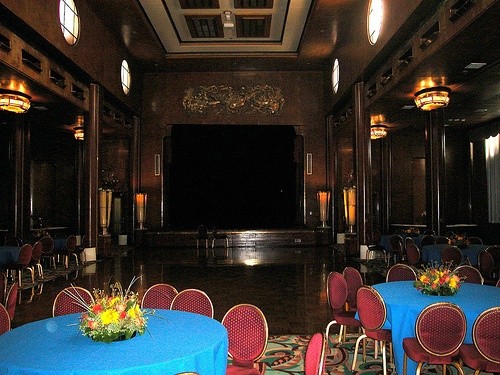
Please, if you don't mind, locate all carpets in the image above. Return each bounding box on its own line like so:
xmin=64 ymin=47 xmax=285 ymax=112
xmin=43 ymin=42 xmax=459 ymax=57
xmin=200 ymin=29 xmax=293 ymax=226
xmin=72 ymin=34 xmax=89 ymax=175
xmin=176 ymin=333 xmax=439 ymax=375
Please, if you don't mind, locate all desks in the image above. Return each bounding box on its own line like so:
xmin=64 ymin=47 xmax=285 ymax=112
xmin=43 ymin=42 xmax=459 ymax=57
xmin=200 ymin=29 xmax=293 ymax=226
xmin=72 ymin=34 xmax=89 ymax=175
xmin=422 ymin=244 xmax=489 ymax=267
xmin=26 ymin=239 xmax=66 ymax=268
xmin=0 ymin=246 xmax=21 ymax=274
xmin=0 ymin=309 xmax=229 ymax=375
xmin=381 ymin=235 xmax=422 ymax=251
xmin=354 ymin=281 xmax=500 ymax=375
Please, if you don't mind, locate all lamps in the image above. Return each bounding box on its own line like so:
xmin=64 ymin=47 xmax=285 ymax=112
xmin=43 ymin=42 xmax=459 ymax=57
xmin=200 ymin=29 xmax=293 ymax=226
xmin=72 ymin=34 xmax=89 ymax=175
xmin=371 ymin=125 xmax=389 ymax=140
xmin=73 ymin=127 xmax=84 ymax=140
xmin=413 ymin=86 xmax=452 ymax=112
xmin=0 ymin=88 xmax=32 ymax=116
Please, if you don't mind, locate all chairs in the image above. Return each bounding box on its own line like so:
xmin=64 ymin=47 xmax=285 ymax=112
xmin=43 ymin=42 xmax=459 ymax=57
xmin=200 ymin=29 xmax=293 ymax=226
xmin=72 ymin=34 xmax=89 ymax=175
xmin=0 ymin=224 xmax=500 ymax=375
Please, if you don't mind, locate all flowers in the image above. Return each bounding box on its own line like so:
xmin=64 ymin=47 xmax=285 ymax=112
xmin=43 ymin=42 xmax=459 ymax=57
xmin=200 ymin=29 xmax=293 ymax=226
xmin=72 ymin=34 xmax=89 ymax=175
xmin=62 ymin=275 xmax=171 ymax=343
xmin=413 ymin=259 xmax=468 ymax=296
